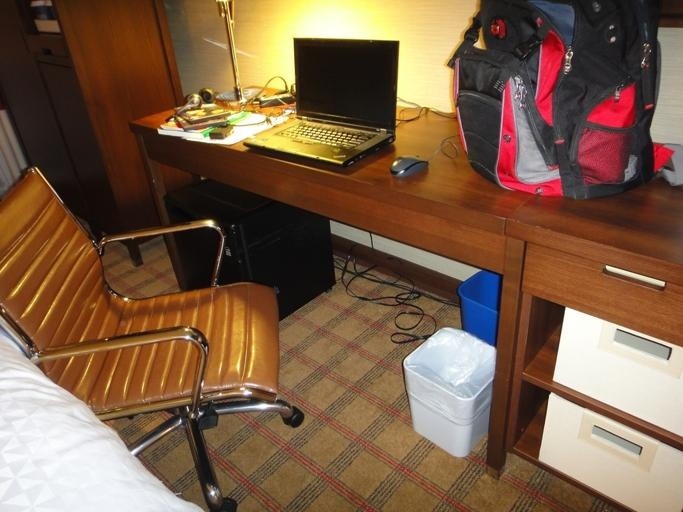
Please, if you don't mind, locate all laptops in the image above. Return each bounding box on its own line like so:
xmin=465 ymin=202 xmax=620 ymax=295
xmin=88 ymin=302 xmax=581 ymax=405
xmin=242 ymin=37 xmax=400 ymax=168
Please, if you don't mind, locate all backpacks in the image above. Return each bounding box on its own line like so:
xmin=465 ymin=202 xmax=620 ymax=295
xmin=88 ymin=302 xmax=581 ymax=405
xmin=448 ymin=0 xmax=674 ymax=199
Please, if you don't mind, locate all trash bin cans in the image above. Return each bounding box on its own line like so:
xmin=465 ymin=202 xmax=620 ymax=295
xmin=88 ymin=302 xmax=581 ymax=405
xmin=403 ymin=327 xmax=497 ymax=457
xmin=458 ymin=270 xmax=501 ymax=346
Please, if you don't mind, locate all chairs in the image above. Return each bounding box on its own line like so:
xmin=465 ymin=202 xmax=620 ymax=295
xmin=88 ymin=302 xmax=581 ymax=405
xmin=0 ymin=167 xmax=304 ymax=510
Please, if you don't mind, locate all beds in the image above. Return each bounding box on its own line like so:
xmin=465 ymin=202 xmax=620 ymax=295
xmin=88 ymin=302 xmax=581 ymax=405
xmin=0 ymin=322 xmax=205 ymax=511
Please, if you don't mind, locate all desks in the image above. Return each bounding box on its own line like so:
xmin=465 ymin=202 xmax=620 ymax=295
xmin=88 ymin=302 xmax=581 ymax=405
xmin=127 ymin=84 xmax=531 ymax=480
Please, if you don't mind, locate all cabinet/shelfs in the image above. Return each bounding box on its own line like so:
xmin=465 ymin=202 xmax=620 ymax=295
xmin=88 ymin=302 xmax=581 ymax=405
xmin=503 ymin=176 xmax=683 ymax=512
xmin=0 ymin=0 xmax=187 ymax=266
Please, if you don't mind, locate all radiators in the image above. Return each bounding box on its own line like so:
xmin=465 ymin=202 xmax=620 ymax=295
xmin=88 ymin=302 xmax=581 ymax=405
xmin=0 ymin=107 xmax=28 ymax=198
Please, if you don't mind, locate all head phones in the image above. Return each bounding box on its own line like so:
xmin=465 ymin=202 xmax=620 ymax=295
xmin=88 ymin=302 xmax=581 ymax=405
xmin=173 ymin=88 xmax=214 ymax=116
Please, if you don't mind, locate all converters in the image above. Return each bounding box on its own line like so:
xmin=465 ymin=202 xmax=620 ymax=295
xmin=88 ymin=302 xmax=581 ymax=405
xmin=258 ymin=92 xmax=293 ymax=108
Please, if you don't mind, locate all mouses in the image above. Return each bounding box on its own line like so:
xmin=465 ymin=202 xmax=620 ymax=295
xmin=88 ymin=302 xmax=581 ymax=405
xmin=389 ymin=154 xmax=429 ymax=176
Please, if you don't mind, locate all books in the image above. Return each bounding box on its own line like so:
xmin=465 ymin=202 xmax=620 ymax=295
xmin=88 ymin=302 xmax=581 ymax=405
xmin=158 ymin=102 xmax=231 ymax=140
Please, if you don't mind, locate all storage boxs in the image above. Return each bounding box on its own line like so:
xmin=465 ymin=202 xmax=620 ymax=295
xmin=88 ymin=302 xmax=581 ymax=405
xmin=538 ymin=388 xmax=682 ymax=512
xmin=551 ymin=306 xmax=683 ymax=438
